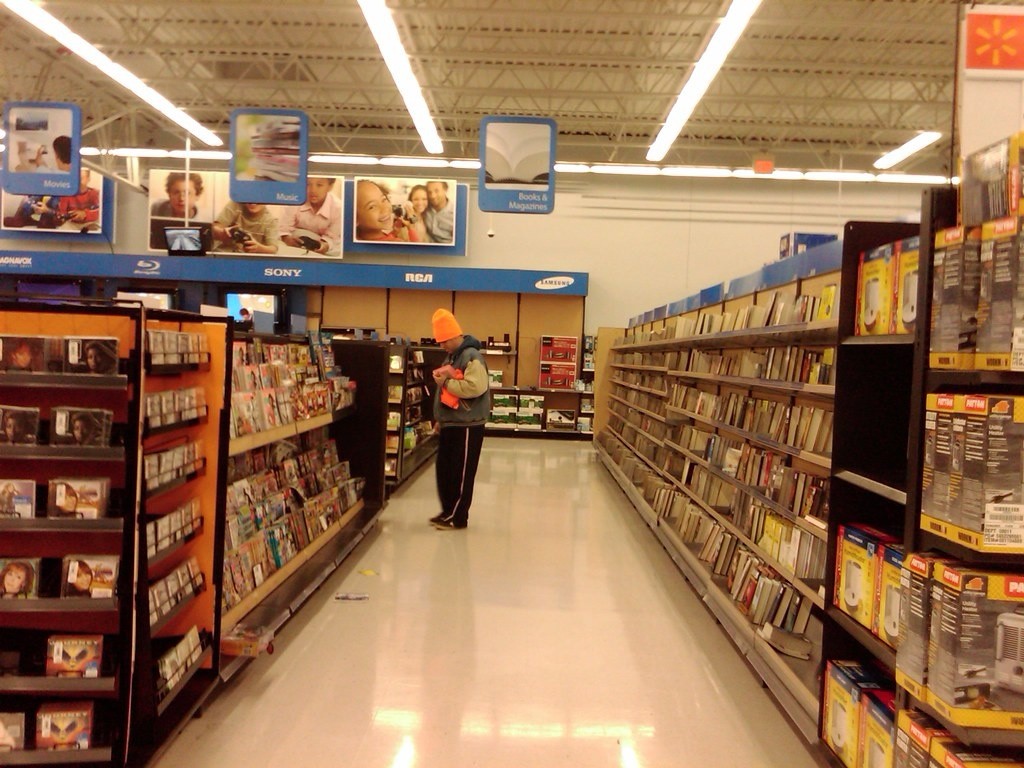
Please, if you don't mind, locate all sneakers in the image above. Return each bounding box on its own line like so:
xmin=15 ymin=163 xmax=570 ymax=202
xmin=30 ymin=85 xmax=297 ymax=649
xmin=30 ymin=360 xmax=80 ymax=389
xmin=430 ymin=513 xmax=467 ymax=530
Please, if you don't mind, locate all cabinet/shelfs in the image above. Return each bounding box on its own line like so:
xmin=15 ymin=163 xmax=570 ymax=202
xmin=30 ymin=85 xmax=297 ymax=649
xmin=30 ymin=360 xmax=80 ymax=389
xmin=589 ymin=183 xmax=1024 ymax=767
xmin=0 ymin=296 xmax=516 ymax=768
xmin=484 ymin=386 xmax=594 ymax=435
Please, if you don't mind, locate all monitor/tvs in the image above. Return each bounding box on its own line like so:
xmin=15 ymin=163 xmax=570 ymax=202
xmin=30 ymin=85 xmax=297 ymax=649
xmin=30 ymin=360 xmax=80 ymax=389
xmin=163 ymin=226 xmax=212 ymax=256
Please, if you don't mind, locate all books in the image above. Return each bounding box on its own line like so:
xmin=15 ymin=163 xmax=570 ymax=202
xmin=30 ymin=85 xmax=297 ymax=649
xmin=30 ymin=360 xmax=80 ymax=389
xmin=597 ymin=283 xmax=840 ymax=659
xmin=220 ymin=328 xmax=433 ymax=618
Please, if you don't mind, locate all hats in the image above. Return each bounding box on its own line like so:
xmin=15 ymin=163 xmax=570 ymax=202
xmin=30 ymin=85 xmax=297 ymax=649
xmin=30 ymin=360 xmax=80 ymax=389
xmin=432 ymin=309 xmax=463 ymax=342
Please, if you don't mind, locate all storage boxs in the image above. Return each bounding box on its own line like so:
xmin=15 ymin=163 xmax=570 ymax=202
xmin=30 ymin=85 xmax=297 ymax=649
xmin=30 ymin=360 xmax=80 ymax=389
xmin=484 ymin=334 xmax=598 ymax=430
xmin=823 ymin=133 xmax=1024 ymax=767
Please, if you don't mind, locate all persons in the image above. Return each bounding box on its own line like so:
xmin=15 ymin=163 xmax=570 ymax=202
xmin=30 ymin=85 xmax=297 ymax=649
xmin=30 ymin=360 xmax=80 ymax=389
xmin=0 ymin=340 xmax=116 ymax=598
xmin=4 ymin=134 xmax=453 ymax=256
xmin=426 ymin=309 xmax=490 ymax=529
